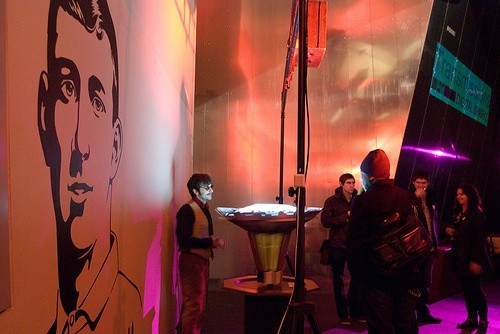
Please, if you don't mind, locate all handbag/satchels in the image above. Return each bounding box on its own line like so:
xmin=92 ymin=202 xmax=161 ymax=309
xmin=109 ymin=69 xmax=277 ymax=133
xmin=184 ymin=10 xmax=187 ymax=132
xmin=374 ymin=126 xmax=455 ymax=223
xmin=481 ymin=239 xmax=495 ymax=269
xmin=359 ymin=189 xmax=433 ymax=276
xmin=319 ymin=239 xmax=330 ymax=266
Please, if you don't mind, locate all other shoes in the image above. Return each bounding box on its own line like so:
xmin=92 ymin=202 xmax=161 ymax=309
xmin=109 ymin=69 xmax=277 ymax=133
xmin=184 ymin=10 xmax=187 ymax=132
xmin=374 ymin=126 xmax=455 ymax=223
xmin=339 ymin=319 xmax=351 ymax=324
xmin=416 ymin=314 xmax=441 ymax=325
xmin=476 ymin=320 xmax=488 ymax=334
xmin=457 ymin=318 xmax=478 ymax=329
xmin=350 ymin=317 xmax=367 ymax=323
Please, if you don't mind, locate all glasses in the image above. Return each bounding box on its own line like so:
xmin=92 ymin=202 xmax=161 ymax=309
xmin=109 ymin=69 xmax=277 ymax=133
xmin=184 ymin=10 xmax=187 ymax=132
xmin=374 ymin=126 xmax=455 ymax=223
xmin=200 ymin=184 xmax=213 ymax=191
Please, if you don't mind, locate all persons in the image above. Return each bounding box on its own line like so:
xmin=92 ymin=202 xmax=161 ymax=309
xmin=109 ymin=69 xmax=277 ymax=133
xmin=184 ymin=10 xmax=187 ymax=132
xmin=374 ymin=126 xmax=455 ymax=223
xmin=405 ymin=174 xmax=440 ymax=323
xmin=347 ymin=148 xmax=424 ymax=334
xmin=445 ymin=183 xmax=491 ymax=330
xmin=321 ymin=174 xmax=368 ymax=325
xmin=176 ymin=173 xmax=224 ymax=334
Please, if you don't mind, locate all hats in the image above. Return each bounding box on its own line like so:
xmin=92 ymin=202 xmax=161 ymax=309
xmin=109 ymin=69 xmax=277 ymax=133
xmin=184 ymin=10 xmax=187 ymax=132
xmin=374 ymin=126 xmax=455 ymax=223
xmin=360 ymin=149 xmax=390 ymax=182
